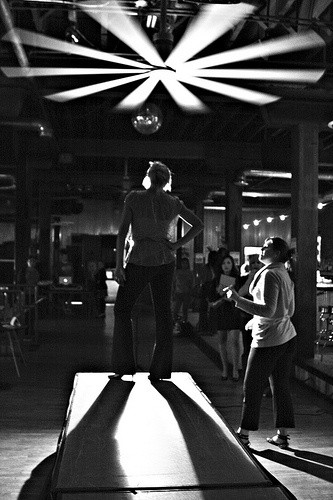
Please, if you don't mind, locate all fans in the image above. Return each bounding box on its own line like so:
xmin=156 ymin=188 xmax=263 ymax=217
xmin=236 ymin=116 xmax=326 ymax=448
xmin=3 ymin=0 xmax=326 ymax=111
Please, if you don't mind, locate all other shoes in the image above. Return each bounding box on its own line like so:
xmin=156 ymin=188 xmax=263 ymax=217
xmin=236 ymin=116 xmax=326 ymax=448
xmin=94 ymin=315 xmax=106 ymax=319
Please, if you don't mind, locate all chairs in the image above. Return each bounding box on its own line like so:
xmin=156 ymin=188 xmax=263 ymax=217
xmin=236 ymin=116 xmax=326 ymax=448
xmin=0 ymin=283 xmax=41 ymax=378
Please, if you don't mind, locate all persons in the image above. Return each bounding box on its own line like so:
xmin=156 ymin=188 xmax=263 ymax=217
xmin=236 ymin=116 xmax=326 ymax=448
xmin=96 ymin=260 xmax=110 ymax=319
xmin=106 ymin=162 xmax=207 ymax=384
xmin=224 ymin=236 xmax=297 ymax=450
xmin=209 ymin=254 xmax=250 ymax=383
xmin=55 ymin=252 xmax=76 ymax=288
xmin=17 ymin=255 xmax=40 ymax=336
xmin=173 ymin=257 xmax=196 ymax=326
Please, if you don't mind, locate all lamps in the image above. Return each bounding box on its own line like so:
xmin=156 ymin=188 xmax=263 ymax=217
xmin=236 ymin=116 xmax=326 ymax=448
xmin=131 ymin=103 xmax=163 ymax=135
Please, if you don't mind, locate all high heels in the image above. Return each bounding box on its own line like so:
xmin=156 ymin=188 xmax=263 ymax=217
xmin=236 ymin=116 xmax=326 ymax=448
xmin=232 ymin=370 xmax=240 ymax=382
xmin=148 ymin=373 xmax=170 ymax=380
xmin=221 ymin=370 xmax=229 ymax=380
xmin=108 ymin=370 xmax=135 ymax=380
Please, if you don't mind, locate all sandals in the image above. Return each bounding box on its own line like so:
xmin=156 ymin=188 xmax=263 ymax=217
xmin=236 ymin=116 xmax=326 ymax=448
xmin=235 ymin=431 xmax=250 ymax=448
xmin=265 ymin=434 xmax=289 ymax=450
